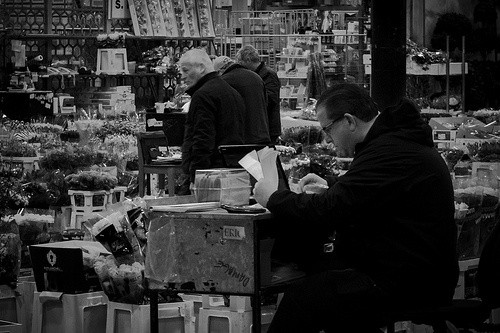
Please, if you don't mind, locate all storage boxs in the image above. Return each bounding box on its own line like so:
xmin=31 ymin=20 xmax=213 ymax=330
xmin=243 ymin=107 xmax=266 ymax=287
xmin=128 ymin=0 xmax=216 ymax=37
xmin=29 ymin=241 xmax=100 ymax=295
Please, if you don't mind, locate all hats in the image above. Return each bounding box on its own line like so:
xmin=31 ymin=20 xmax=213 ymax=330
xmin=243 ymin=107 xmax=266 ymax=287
xmin=213 ymin=56 xmax=232 ymax=72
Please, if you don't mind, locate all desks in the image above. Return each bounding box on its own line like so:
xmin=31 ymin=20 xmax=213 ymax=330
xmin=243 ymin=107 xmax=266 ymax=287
xmin=142 ymin=201 xmax=306 ymax=333
xmin=364 ymin=62 xmax=468 ymax=114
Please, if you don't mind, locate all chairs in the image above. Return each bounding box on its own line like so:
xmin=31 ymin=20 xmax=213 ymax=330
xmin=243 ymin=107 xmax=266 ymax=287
xmin=385 ymin=221 xmax=500 ymax=333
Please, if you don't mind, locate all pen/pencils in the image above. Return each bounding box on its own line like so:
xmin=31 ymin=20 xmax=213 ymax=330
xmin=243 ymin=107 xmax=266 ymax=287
xmin=288 ymin=176 xmax=328 ymax=188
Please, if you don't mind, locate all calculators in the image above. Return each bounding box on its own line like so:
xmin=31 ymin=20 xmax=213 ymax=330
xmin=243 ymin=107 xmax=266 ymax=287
xmin=221 ymin=204 xmax=265 ymax=214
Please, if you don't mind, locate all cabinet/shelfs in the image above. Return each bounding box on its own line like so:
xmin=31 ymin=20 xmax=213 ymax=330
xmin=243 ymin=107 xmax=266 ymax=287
xmin=275 ymin=53 xmax=314 ymax=109
xmin=120 ymin=36 xmax=218 ymax=103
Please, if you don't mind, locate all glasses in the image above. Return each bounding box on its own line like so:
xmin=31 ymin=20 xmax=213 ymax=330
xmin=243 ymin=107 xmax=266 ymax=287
xmin=320 ymin=114 xmax=346 ymax=139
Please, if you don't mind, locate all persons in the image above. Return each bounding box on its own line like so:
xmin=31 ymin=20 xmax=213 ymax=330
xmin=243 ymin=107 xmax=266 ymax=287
xmin=212 ymin=56 xmax=272 ymax=166
xmin=174 ymin=48 xmax=245 ymax=196
xmin=235 ymin=45 xmax=282 ymax=144
xmin=252 ymin=82 xmax=461 ymax=333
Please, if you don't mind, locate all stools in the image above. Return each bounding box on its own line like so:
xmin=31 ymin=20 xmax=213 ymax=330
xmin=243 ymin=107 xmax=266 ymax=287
xmin=136 ymin=132 xmax=182 ymax=197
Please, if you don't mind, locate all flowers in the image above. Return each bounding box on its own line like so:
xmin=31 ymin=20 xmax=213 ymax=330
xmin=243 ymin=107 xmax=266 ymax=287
xmin=0 ymin=33 xmax=500 ymax=303
